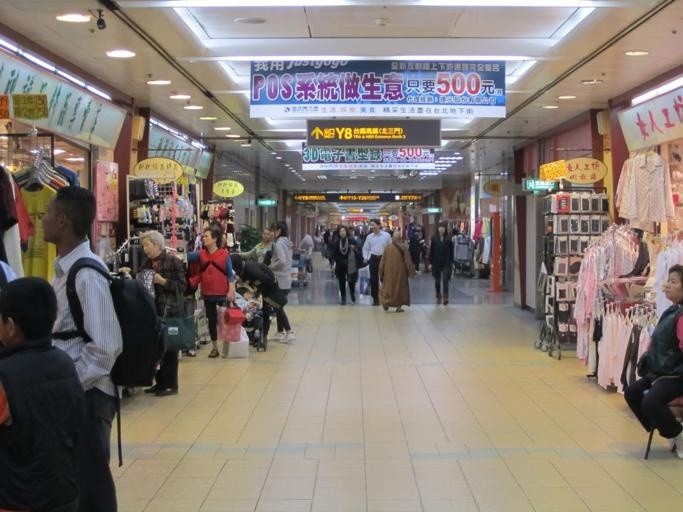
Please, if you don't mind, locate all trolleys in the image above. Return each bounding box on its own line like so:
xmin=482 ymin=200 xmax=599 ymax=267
xmin=291 ymin=249 xmax=310 ymax=287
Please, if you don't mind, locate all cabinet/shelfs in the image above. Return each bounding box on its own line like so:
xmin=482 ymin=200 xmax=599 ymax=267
xmin=533 ymin=186 xmax=612 ymax=359
xmin=126 ymin=175 xmax=204 ymax=360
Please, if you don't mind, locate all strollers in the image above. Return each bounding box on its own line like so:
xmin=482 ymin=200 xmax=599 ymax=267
xmin=233 ymin=280 xmax=267 ymax=352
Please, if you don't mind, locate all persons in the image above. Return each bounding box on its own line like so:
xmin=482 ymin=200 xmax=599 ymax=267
xmin=324 ymin=218 xmax=455 ymax=313
xmin=0 ymin=276 xmax=85 ymax=512
xmin=236 ymin=287 xmax=254 ymax=322
xmin=42 ymin=188 xmax=124 ymax=512
xmin=231 ymin=254 xmax=288 ymax=347
xmin=118 ymin=230 xmax=188 ymax=397
xmin=229 ymin=228 xmax=273 ymax=263
xmin=187 ymin=227 xmax=235 ymax=359
xmin=267 ymin=221 xmax=295 ymax=343
xmin=624 ymin=264 xmax=683 ymax=460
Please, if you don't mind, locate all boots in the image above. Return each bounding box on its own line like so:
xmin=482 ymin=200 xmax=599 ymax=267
xmin=437 ymin=293 xmax=442 ymax=303
xmin=342 ymin=296 xmax=346 ymax=304
xmin=443 ymin=293 xmax=448 ymax=305
xmin=351 ymin=293 xmax=355 ymax=302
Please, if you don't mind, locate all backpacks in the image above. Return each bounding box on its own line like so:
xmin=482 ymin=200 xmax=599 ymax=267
xmin=67 ymin=257 xmax=166 ymax=387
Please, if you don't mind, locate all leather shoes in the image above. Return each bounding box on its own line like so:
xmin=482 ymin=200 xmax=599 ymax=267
xmin=144 ymin=384 xmax=159 ymax=393
xmin=156 ymin=386 xmax=178 ymax=395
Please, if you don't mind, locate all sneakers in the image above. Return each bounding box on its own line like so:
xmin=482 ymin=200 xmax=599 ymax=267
xmin=396 ymin=308 xmax=404 ymax=312
xmin=668 ymin=433 xmax=683 ymax=453
xmin=675 ymin=431 xmax=683 ymax=459
xmin=280 ymin=331 xmax=295 ymax=343
xmin=187 ymin=349 xmax=196 ymax=356
xmin=208 ymin=349 xmax=219 ymax=358
xmin=383 ymin=305 xmax=388 ymax=310
xmin=270 ymin=332 xmax=285 ymax=341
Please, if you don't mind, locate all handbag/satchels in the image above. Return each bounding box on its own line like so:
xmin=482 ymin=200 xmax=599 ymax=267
xmin=224 ymin=302 xmax=245 ymax=325
xmin=216 ymin=324 xmax=249 ymax=357
xmin=216 ymin=305 xmax=241 ymax=342
xmin=155 ymin=315 xmax=196 ymax=352
xmin=348 ymin=250 xmax=356 ymax=276
xmin=637 ymin=352 xmax=650 ymax=378
xmin=307 ymin=259 xmax=312 ymax=272
xmin=264 ymin=285 xmax=288 ymax=314
xmin=358 ymin=264 xmax=370 ymax=296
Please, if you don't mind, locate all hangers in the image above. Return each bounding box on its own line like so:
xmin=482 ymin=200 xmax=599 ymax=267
xmin=21 ymin=146 xmax=53 ymax=191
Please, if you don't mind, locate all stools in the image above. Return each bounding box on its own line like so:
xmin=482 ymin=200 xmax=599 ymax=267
xmin=645 ymin=396 xmax=683 ymax=460
